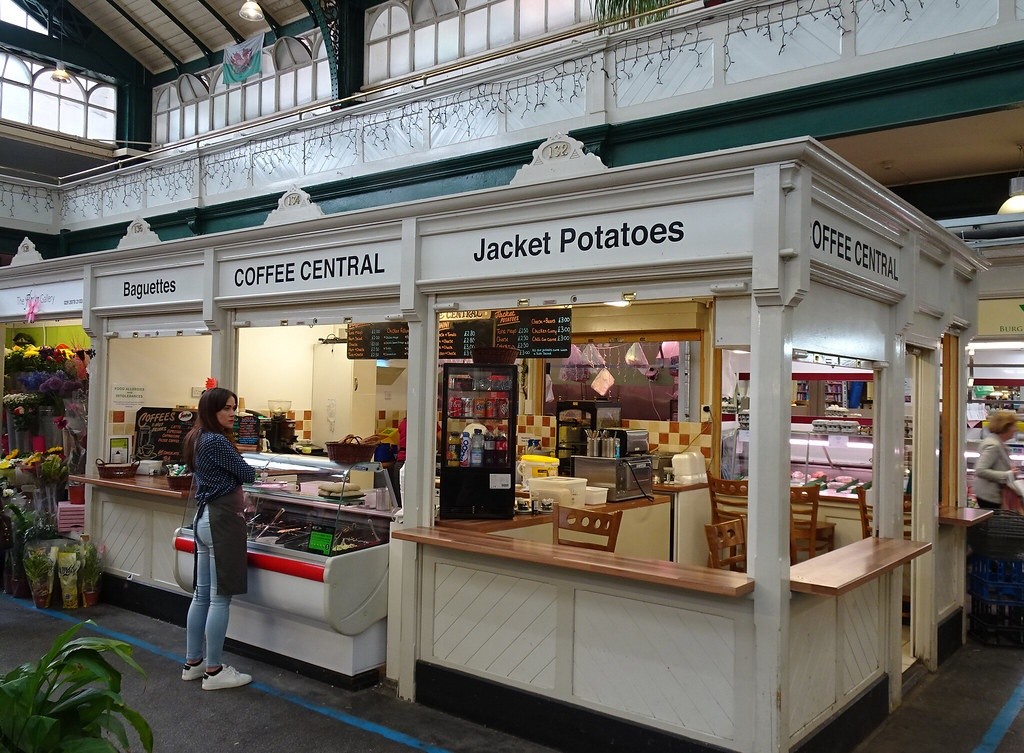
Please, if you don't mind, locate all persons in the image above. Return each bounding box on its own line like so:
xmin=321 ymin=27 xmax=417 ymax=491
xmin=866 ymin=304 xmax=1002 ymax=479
xmin=179 ymin=388 xmax=256 ymax=690
xmin=972 ymin=411 xmax=1019 ymax=513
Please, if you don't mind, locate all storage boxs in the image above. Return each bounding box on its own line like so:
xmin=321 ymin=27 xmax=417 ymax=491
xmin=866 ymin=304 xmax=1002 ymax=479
xmin=586 ymin=487 xmax=609 ymax=504
xmin=528 ymin=476 xmax=587 ymax=508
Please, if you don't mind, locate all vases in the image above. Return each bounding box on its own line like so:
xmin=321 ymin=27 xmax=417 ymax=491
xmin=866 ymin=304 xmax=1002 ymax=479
xmin=69 ymin=485 xmax=84 ymax=504
xmin=11 ymin=578 xmax=27 ymax=597
xmin=22 ymin=547 xmax=52 ymax=608
xmin=4 ymin=575 xmax=14 ymax=594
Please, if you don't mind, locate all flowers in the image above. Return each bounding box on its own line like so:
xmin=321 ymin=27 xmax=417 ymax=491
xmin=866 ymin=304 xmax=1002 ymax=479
xmin=0 ymin=342 xmax=94 ymax=542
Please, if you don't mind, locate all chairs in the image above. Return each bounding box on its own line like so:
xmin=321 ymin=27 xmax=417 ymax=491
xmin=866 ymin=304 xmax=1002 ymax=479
xmin=553 ymin=502 xmax=623 ymax=552
xmin=704 ymin=471 xmax=912 ymax=616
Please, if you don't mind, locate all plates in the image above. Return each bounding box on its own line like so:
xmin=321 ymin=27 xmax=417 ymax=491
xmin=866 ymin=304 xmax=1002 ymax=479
xmin=515 ymin=508 xmax=531 ymax=515
xmin=538 ymin=509 xmax=553 ymax=515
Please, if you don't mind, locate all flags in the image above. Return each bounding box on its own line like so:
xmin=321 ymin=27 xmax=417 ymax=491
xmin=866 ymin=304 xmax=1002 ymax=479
xmin=222 ymin=33 xmax=264 ymax=84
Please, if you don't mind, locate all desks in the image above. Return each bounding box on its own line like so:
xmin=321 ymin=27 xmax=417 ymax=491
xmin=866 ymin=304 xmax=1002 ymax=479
xmin=69 ymin=473 xmax=194 ymax=498
xmin=938 ymin=507 xmax=994 ymax=526
xmin=790 ymin=536 xmax=933 ymax=595
xmin=391 ymin=522 xmax=753 ymax=594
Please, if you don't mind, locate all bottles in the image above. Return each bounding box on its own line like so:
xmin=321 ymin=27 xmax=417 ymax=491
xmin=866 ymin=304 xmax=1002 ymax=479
xmin=113 ymin=451 xmax=121 ymax=463
xmin=260 ymin=435 xmax=267 ymax=452
xmin=528 ymin=439 xmax=541 ymax=451
xmin=531 ymin=492 xmax=539 ymax=515
xmin=447 ymin=428 xmax=508 ymax=467
xmin=376 ymin=488 xmax=391 ymax=511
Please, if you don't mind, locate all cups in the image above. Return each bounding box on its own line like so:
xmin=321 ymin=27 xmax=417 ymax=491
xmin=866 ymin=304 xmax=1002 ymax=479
xmin=587 ymin=437 xmax=602 ymax=457
xmin=602 ymin=438 xmax=620 ymax=457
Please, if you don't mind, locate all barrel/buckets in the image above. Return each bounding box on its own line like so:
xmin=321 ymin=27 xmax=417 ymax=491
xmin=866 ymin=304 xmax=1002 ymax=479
xmin=517 ymin=455 xmax=560 ymax=488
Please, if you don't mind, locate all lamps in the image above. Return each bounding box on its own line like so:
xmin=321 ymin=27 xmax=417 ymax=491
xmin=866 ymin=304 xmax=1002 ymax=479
xmin=990 ymin=385 xmax=1004 ymax=396
xmin=50 ymin=0 xmax=71 ymax=83
xmin=239 ymin=0 xmax=265 ymax=21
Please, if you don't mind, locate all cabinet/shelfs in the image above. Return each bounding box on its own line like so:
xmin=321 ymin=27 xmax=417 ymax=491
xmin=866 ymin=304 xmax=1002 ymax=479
xmin=797 ymin=380 xmax=847 ymax=409
xmin=557 ymin=400 xmax=622 ymax=478
xmin=440 ymin=363 xmax=518 ymax=521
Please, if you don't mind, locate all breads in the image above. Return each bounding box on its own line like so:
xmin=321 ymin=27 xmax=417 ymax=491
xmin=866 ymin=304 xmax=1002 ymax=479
xmin=318 ymin=481 xmax=364 ymax=497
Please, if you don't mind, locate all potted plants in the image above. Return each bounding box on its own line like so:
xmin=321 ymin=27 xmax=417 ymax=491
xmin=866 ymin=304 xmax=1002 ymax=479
xmin=82 ymin=544 xmax=103 ymax=606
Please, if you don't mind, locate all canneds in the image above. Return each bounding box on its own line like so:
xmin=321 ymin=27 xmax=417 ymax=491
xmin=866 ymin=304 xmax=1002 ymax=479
xmin=797 ymin=394 xmax=800 ymax=401
xmin=448 ymin=397 xmax=510 ymax=418
xmin=833 ymin=385 xmax=841 ymax=402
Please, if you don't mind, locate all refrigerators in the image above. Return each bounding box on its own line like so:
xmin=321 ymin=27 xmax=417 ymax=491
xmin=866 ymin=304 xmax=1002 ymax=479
xmin=440 ymin=363 xmax=517 ymax=521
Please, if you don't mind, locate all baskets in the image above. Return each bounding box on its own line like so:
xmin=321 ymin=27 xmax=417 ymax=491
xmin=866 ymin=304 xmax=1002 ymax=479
xmin=165 ymin=469 xmax=194 ymax=490
xmin=95 ymin=459 xmax=140 ymax=480
xmin=325 ymin=435 xmax=376 ymax=463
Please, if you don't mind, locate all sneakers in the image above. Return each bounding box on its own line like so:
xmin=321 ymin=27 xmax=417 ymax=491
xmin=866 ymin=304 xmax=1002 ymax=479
xmin=182 ymin=658 xmax=208 ymax=680
xmin=201 ymin=663 xmax=251 ymax=690
